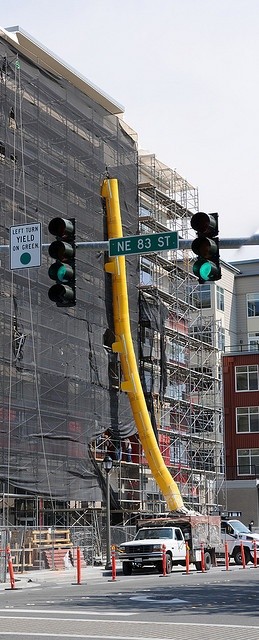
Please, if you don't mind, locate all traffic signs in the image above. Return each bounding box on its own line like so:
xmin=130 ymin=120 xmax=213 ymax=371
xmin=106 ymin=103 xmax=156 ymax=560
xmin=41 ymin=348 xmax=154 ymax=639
xmin=9 ymin=222 xmax=40 ymax=268
xmin=108 ymin=231 xmax=179 ymax=256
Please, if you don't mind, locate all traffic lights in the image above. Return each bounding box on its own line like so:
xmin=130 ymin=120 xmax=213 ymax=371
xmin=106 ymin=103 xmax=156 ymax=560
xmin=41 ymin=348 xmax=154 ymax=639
xmin=48 ymin=217 xmax=76 ymax=307
xmin=191 ymin=212 xmax=221 ymax=284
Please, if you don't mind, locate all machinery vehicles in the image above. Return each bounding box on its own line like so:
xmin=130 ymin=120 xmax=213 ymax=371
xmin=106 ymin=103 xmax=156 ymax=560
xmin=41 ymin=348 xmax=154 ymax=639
xmin=214 ymin=519 xmax=259 ymax=565
xmin=117 ymin=516 xmax=221 ymax=575
